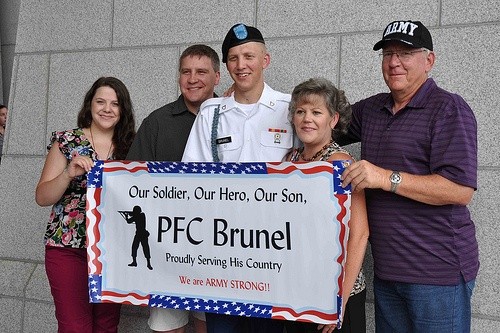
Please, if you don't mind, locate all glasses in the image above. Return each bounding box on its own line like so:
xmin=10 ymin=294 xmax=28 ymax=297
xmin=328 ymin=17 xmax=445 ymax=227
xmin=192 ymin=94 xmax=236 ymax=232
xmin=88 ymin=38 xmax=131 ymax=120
xmin=379 ymin=49 xmax=427 ymax=61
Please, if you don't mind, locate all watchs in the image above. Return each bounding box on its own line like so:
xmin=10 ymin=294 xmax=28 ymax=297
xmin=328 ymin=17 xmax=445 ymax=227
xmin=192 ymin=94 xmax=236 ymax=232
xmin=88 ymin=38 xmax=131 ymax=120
xmin=389 ymin=170 xmax=402 ymax=193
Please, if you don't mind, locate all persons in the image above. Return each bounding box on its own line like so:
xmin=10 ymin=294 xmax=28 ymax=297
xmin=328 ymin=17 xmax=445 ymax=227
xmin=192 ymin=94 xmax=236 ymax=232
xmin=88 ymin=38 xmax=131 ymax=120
xmin=269 ymin=77 xmax=369 ymax=333
xmin=331 ymin=19 xmax=481 ymax=333
xmin=124 ymin=44 xmax=223 ymax=332
xmin=33 ymin=76 xmax=136 ymax=333
xmin=180 ymin=23 xmax=304 ymax=333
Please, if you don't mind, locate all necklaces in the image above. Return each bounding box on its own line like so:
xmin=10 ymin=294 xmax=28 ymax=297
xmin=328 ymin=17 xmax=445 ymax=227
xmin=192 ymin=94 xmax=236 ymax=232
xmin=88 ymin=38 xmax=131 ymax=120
xmin=89 ymin=127 xmax=113 ymax=160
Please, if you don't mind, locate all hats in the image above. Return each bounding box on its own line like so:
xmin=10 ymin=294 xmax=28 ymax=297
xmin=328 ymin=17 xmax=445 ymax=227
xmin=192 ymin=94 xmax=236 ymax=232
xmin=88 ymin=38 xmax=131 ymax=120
xmin=373 ymin=20 xmax=433 ymax=51
xmin=222 ymin=23 xmax=265 ymax=63
